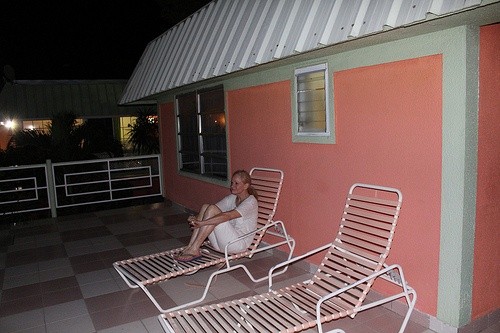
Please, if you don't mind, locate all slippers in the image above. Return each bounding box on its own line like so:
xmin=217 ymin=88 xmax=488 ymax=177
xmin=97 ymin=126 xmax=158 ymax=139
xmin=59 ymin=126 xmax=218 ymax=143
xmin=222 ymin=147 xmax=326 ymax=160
xmin=174 ymin=247 xmax=202 ymax=261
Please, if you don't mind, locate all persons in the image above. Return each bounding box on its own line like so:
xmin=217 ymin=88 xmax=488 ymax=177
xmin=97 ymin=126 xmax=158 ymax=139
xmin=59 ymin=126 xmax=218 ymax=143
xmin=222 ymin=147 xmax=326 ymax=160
xmin=174 ymin=169 xmax=259 ymax=261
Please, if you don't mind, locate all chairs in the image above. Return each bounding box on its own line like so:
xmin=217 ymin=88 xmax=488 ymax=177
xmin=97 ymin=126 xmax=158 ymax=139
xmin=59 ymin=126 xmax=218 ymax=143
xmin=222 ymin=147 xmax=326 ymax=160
xmin=113 ymin=167 xmax=418 ymax=333
